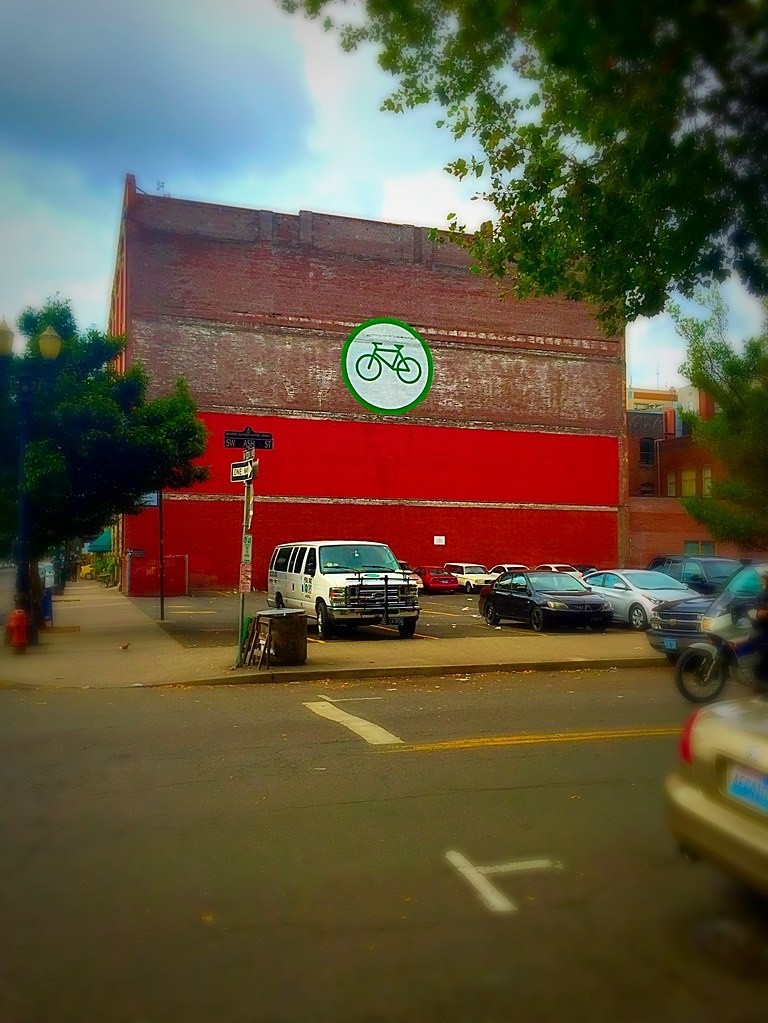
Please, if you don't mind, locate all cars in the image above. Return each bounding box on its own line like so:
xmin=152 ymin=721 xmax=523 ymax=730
xmin=578 ymin=569 xmax=700 ymax=631
xmin=530 ymin=564 xmax=584 ymax=590
xmin=478 ymin=570 xmax=614 ymax=632
xmin=38 ymin=558 xmax=64 ymax=591
xmin=646 ymin=562 xmax=768 ymax=664
xmin=397 ymin=561 xmax=425 ymax=590
xmin=664 ymin=689 xmax=768 ymax=896
xmin=441 ymin=563 xmax=501 ymax=594
xmin=489 ymin=565 xmax=530 ymax=578
xmin=80 ymin=564 xmax=95 ymax=580
xmin=414 ymin=566 xmax=459 ymax=594
xmin=647 ymin=554 xmax=744 ymax=595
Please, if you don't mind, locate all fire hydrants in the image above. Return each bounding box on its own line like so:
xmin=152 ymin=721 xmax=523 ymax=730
xmin=6 ymin=610 xmax=28 ymax=654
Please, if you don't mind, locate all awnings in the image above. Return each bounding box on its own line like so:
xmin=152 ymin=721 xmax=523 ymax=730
xmin=87 ymin=528 xmax=111 ymax=552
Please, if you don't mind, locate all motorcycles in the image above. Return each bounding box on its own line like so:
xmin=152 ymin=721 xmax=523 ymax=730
xmin=674 ymin=565 xmax=768 ymax=703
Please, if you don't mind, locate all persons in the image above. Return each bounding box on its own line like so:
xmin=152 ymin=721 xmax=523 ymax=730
xmin=749 ymin=563 xmax=768 ymax=683
xmin=69 ymin=557 xmax=78 ymax=582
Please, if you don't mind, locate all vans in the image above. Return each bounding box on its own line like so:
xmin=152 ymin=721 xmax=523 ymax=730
xmin=266 ymin=540 xmax=423 ymax=640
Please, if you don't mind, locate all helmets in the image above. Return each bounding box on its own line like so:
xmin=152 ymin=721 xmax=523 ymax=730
xmin=755 ymin=563 xmax=768 ymax=578
